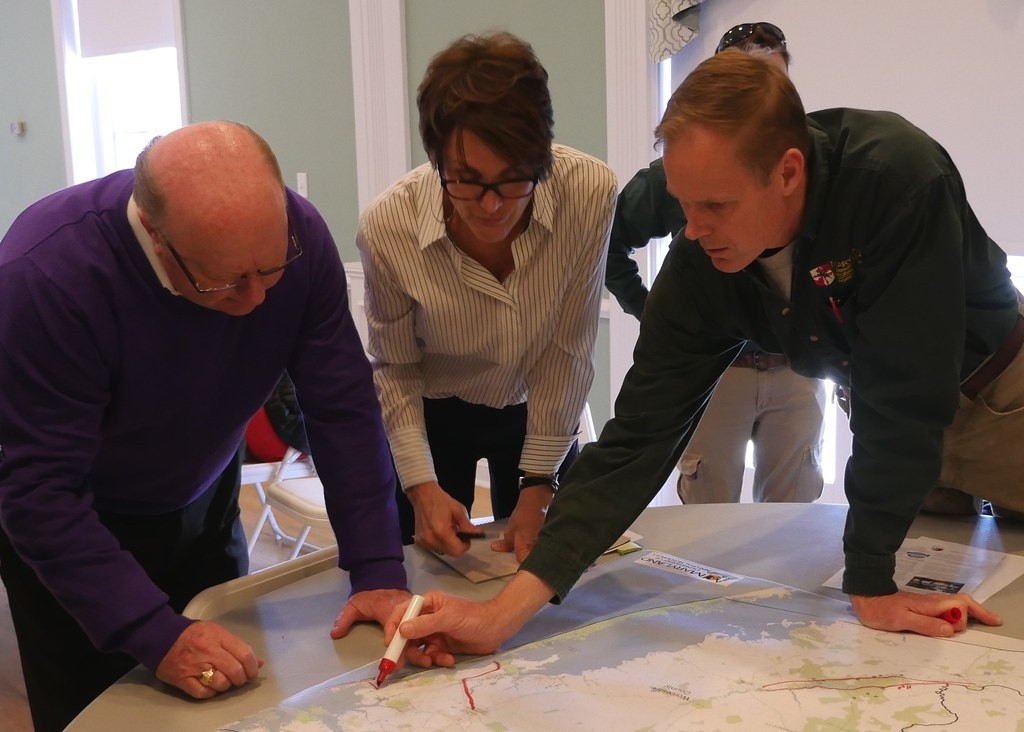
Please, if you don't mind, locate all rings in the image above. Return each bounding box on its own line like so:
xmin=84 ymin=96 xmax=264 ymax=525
xmin=435 ymin=548 xmax=444 ymax=555
xmin=200 ymin=668 xmax=217 ymax=687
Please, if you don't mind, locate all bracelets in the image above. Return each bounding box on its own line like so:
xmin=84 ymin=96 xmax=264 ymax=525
xmin=519 ymin=476 xmax=559 ymax=494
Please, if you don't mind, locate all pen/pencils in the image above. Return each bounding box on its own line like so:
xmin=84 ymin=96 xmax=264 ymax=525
xmin=829 ymin=296 xmax=843 ymax=321
xmin=410 ymin=531 xmax=501 ymax=539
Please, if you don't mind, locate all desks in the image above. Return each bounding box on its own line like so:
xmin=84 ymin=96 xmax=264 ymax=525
xmin=64 ymin=502 xmax=1024 ymax=732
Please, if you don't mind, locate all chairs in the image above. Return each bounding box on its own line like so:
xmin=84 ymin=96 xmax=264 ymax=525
xmin=243 ymin=446 xmax=331 ymax=562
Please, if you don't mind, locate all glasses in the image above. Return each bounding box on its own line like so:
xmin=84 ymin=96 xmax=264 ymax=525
xmin=152 ymin=211 xmax=303 ymax=295
xmin=436 ymin=152 xmax=538 ymax=201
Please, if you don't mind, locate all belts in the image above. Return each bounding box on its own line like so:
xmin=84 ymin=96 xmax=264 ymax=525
xmin=727 ymin=348 xmax=787 ymax=373
xmin=959 ymin=313 xmax=1024 ymax=401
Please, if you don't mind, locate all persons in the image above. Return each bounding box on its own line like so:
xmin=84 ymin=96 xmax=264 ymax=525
xmin=384 ymin=52 xmax=1024 ymax=669
xmin=355 ymin=33 xmax=618 ymax=565
xmin=0 ymin=120 xmax=414 ymax=732
xmin=604 ymin=22 xmax=824 ymax=504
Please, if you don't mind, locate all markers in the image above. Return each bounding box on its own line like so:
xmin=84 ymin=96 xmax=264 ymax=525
xmin=375 ymin=594 xmax=426 ymax=688
